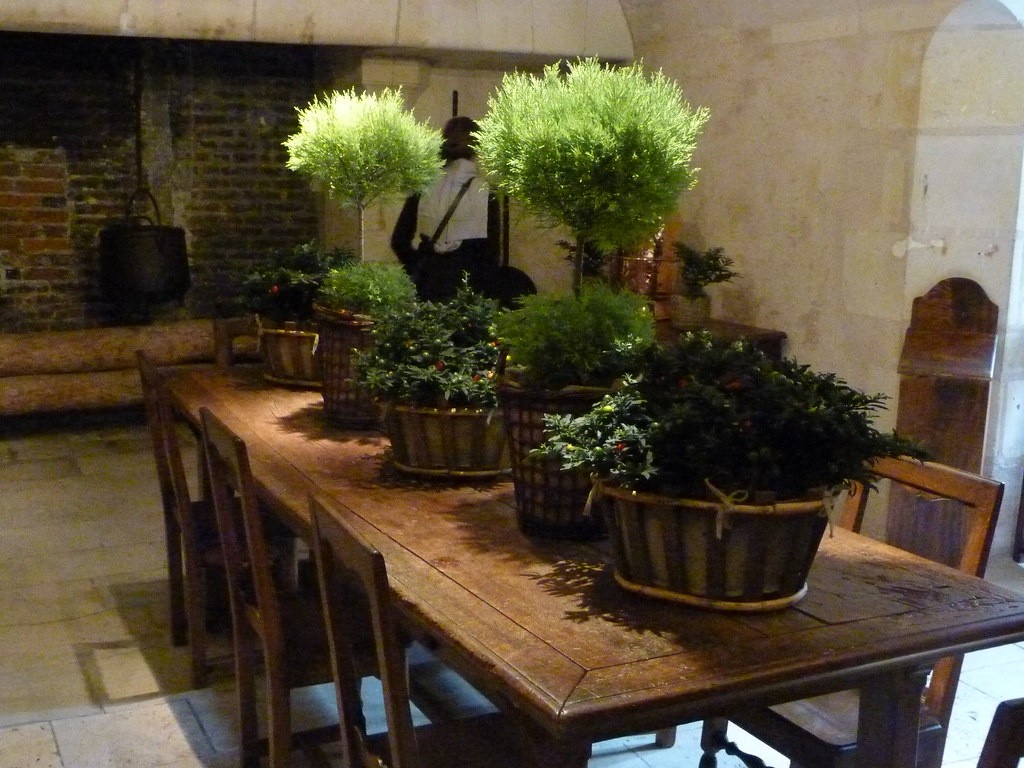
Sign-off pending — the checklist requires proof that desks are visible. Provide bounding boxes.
[156,363,1024,768]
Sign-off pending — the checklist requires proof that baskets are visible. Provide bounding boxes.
[98,189,191,311]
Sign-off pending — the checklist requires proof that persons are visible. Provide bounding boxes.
[390,116,501,289]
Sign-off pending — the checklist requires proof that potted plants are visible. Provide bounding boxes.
[667,241,738,334]
[246,250,356,389]
[353,278,514,487]
[280,88,448,433]
[559,223,670,321]
[528,335,934,613]
[468,58,713,546]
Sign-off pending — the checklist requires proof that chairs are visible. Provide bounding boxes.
[305,488,589,768]
[197,405,408,768]
[214,319,273,366]
[135,348,302,690]
[700,455,1007,768]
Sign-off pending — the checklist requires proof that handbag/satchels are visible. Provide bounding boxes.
[409,235,443,301]
